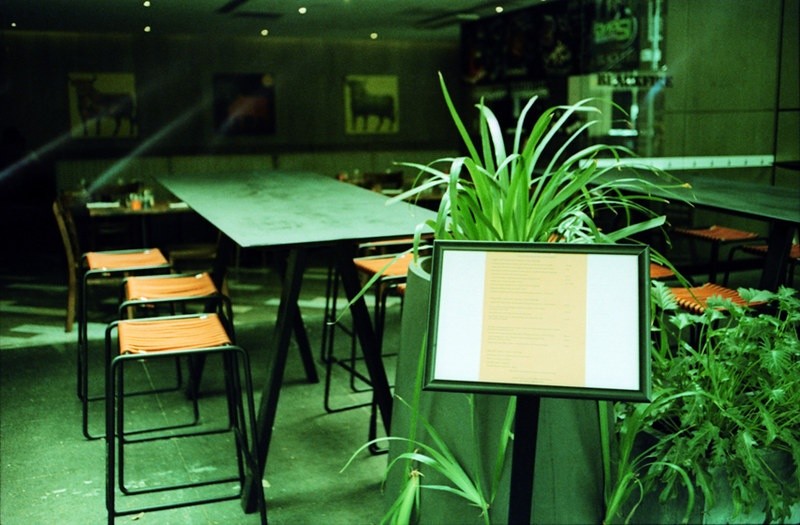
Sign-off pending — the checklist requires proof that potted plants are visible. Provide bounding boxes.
[337,71,704,525]
[613,276,800,525]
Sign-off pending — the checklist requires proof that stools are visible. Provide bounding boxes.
[719,242,800,321]
[321,247,420,456]
[77,246,200,439]
[104,312,269,525]
[649,262,700,355]
[117,269,257,497]
[673,223,768,286]
[651,280,770,373]
[317,234,427,395]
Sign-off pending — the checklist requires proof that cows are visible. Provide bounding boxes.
[343,75,397,133]
[70,73,138,138]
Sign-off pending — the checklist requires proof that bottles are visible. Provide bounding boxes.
[143,189,151,210]
[132,194,142,211]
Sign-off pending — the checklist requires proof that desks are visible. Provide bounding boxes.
[85,186,196,317]
[147,164,463,513]
[356,175,449,211]
[532,163,800,323]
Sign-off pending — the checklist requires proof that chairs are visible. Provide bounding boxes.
[53,198,140,334]
